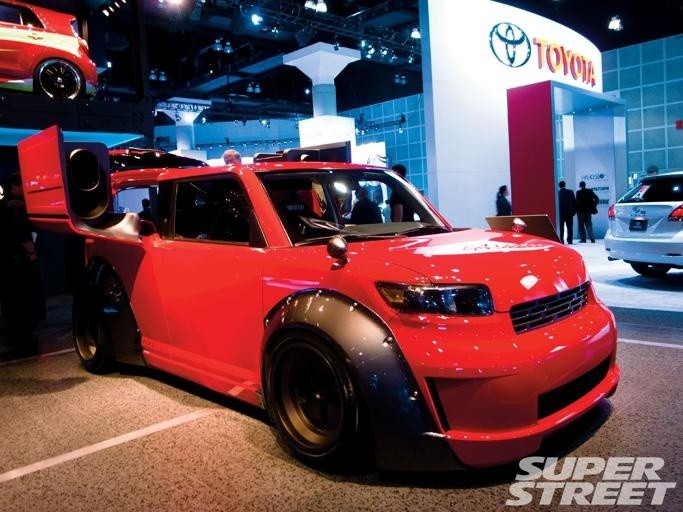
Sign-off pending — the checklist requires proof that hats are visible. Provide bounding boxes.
[18,124,619,469]
[0,1,97,104]
[604,171,683,276]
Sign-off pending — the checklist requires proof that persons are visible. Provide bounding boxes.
[0,170,40,357]
[496,186,511,216]
[559,181,576,244]
[350,187,382,224]
[383,200,392,222]
[389,164,414,222]
[576,182,599,243]
[138,199,151,218]
[223,149,241,165]
[646,166,658,177]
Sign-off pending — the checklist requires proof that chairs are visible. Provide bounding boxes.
[579,238,595,243]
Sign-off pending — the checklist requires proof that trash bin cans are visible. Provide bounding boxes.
[28,251,35,256]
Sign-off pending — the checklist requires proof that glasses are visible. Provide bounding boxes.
[175,111,180,122]
[147,39,262,94]
[304,0,327,14]
[411,28,421,40]
[395,74,408,84]
[334,41,342,50]
